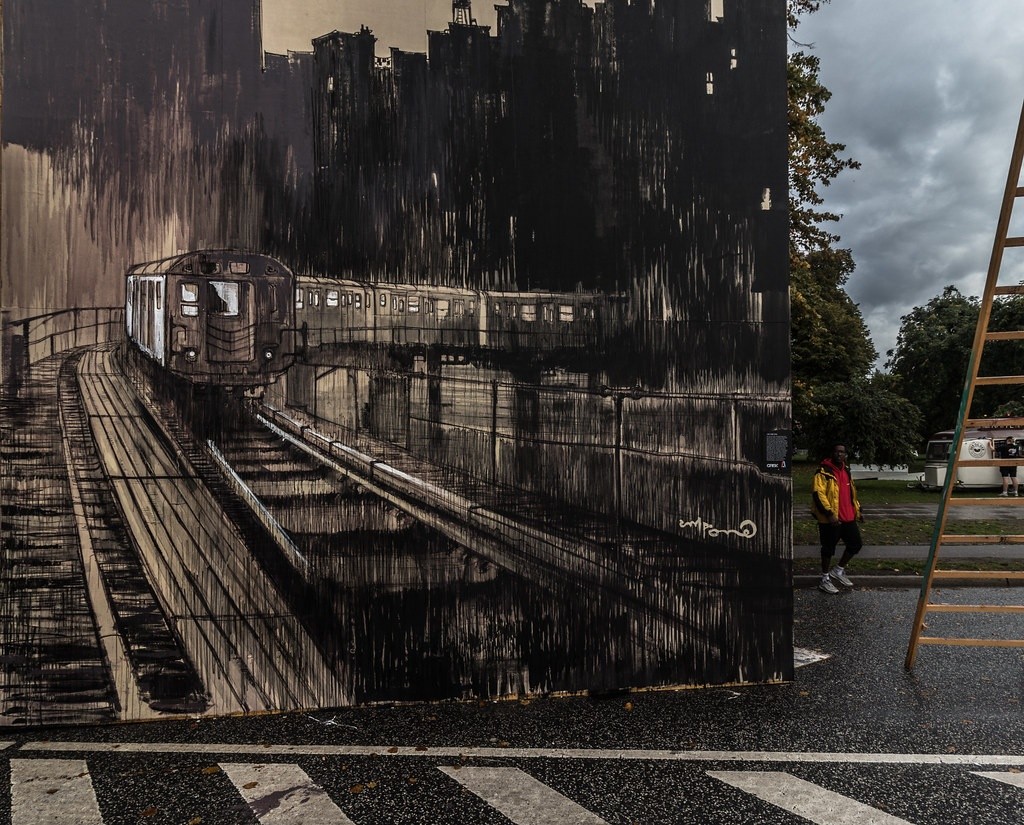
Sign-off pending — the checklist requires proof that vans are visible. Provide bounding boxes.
[922,427,1024,489]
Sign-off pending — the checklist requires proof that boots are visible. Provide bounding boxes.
[828,562,854,586]
[818,573,839,594]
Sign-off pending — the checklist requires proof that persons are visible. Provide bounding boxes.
[987,435,1022,497]
[810,441,866,596]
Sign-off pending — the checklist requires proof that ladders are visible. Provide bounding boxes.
[900,101,1024,674]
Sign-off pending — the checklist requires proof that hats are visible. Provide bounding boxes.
[1006,436,1014,440]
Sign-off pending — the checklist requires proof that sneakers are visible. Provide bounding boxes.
[997,492,1008,496]
[1014,491,1018,496]
